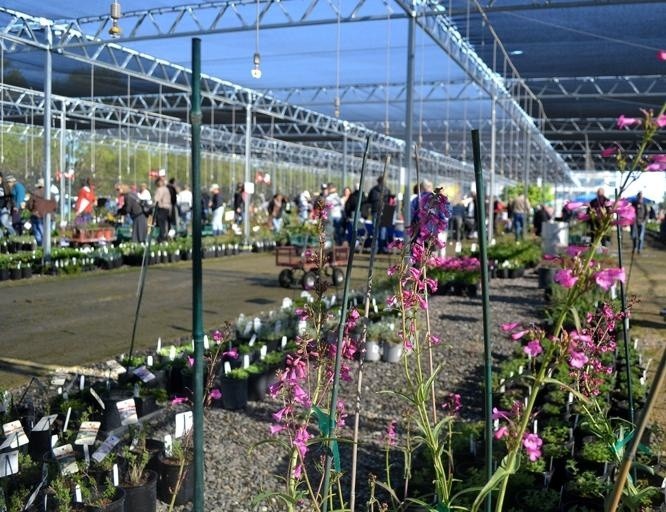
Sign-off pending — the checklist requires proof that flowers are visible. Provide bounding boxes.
[163,328,240,509]
[263,48,664,511]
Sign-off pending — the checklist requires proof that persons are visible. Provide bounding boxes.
[1,171,352,255]
[344,176,551,255]
[561,187,665,256]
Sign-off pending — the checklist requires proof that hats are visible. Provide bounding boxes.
[434,186,443,193]
[320,184,328,190]
[34,178,45,187]
[209,183,219,192]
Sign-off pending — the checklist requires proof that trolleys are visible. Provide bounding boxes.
[274,241,350,290]
[63,225,117,251]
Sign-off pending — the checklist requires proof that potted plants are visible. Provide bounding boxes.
[203,290,404,412]
[2,200,325,281]
[3,340,194,510]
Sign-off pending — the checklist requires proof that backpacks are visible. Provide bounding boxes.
[126,192,153,218]
